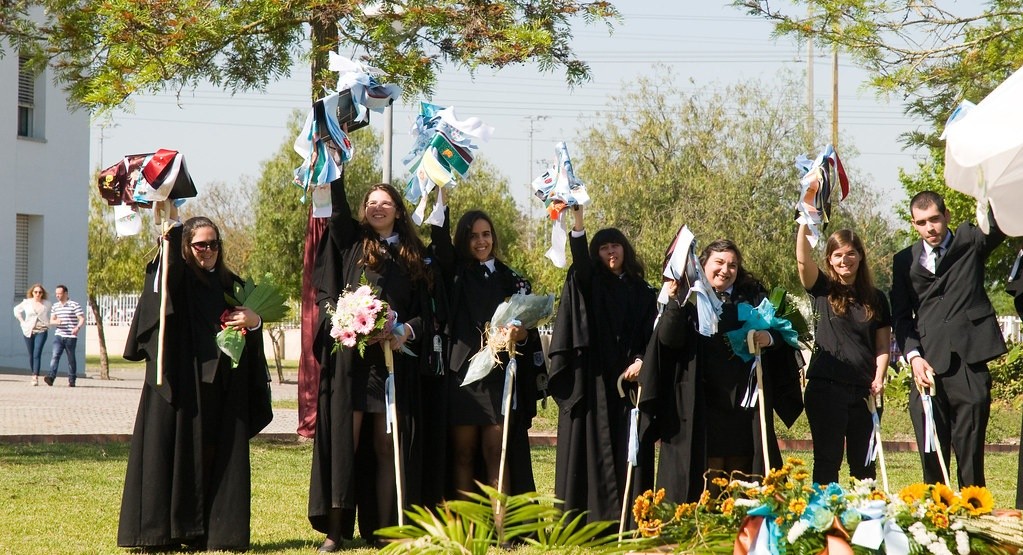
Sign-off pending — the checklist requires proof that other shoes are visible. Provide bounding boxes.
[31,379,39,386]
[69,382,75,387]
[141,543,182,553]
[44,376,54,386]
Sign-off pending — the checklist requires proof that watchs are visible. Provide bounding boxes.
[76,326,80,329]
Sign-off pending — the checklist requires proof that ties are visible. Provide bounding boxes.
[933,247,943,261]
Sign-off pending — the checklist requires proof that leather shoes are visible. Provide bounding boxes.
[366,537,390,549]
[317,532,344,552]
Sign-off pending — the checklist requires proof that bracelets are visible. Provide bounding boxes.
[247,315,261,331]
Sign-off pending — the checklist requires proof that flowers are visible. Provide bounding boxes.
[323,268,419,361]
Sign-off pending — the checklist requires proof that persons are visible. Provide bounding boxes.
[629,240,813,539]
[308,121,433,552]
[14,283,52,386]
[547,203,656,542]
[891,191,1009,492]
[1005,241,1023,510]
[44,285,85,387]
[796,174,891,485]
[117,198,274,551]
[434,184,548,549]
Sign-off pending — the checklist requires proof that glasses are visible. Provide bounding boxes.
[33,290,43,295]
[188,240,222,252]
[365,200,398,209]
